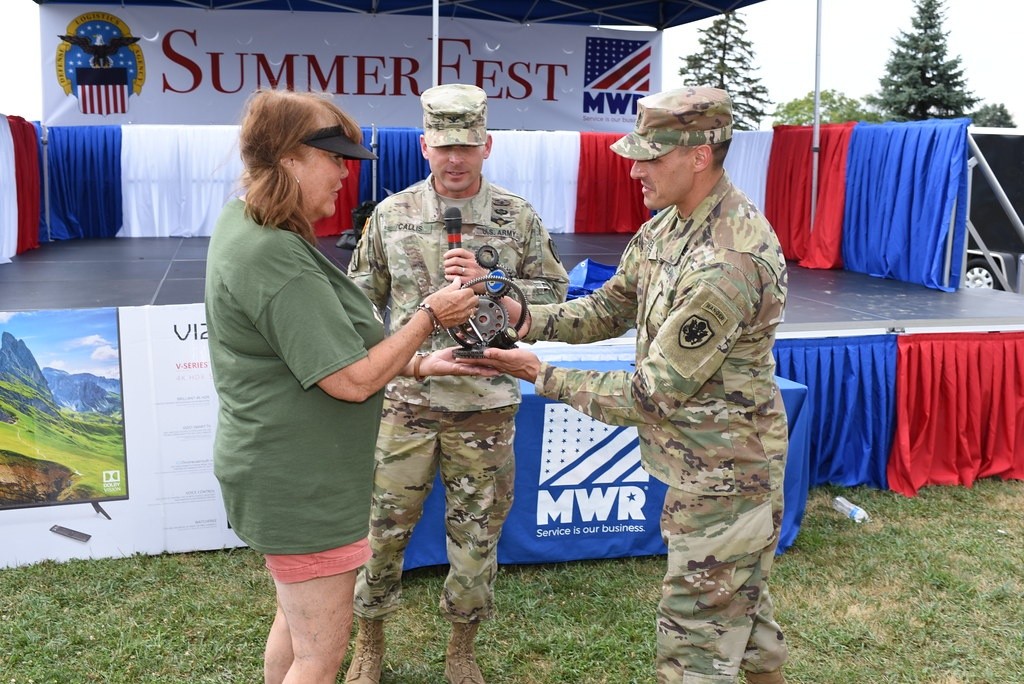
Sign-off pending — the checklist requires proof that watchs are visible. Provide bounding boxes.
[412,350,429,383]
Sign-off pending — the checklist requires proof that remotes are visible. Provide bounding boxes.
[50,525,91,542]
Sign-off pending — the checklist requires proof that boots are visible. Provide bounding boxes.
[344,616,384,684]
[444,621,486,684]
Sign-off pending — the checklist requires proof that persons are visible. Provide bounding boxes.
[339,81,569,684]
[453,85,791,684]
[203,86,500,684]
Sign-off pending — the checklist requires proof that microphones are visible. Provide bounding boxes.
[444,207,463,288]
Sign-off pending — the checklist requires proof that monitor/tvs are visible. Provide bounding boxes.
[0,307,129,520]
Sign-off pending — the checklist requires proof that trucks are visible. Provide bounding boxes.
[963,124,1023,296]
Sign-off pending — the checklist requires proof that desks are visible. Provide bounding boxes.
[405,361,807,573]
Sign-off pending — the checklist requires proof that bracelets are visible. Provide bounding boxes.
[417,302,447,345]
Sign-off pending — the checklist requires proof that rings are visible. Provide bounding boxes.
[462,268,465,273]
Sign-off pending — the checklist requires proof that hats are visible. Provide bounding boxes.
[609,85,736,161]
[299,123,380,160]
[421,84,488,147]
